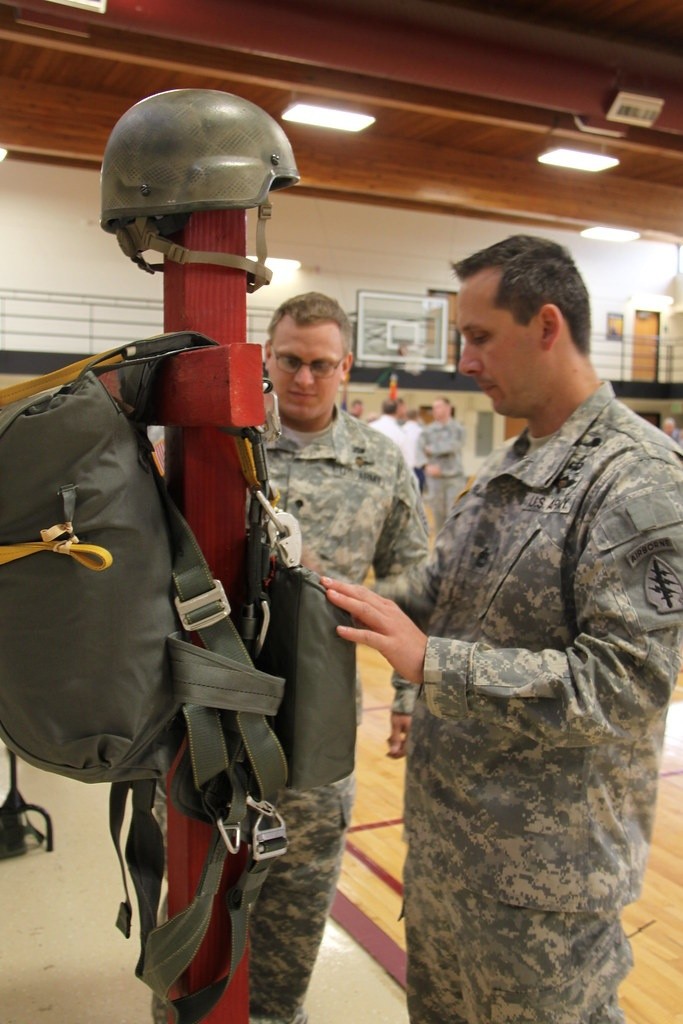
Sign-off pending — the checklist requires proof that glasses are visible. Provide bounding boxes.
[268,342,350,379]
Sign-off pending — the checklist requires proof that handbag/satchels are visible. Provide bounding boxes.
[267,551,358,791]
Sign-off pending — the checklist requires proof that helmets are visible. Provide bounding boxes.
[99,87,304,237]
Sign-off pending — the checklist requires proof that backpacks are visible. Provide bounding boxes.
[0,330,289,1024]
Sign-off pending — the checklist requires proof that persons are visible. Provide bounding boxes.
[314,230,683,1024]
[246,291,433,1024]
[344,393,466,529]
[661,415,683,447]
